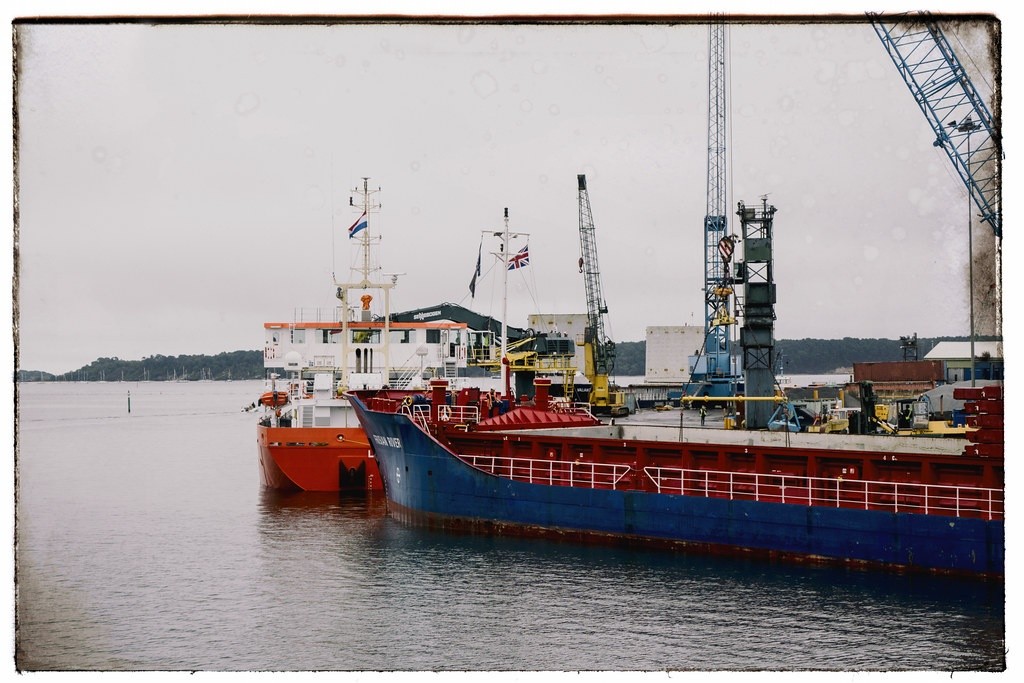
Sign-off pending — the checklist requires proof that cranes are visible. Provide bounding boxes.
[680,19,746,412]
[870,19,999,237]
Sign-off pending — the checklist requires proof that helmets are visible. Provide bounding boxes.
[491,386,496,390]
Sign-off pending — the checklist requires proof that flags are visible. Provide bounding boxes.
[506,244,529,271]
[348,210,368,240]
[469,241,481,298]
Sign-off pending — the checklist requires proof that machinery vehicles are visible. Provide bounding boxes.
[577,173,630,417]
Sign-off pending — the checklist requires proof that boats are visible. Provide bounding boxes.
[343,191,1004,595]
[244,176,519,493]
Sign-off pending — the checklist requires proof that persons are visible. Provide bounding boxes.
[699,405,706,426]
[901,405,912,428]
[481,387,504,418]
[780,411,787,431]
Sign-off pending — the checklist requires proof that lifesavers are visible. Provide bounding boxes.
[440,407,452,422]
